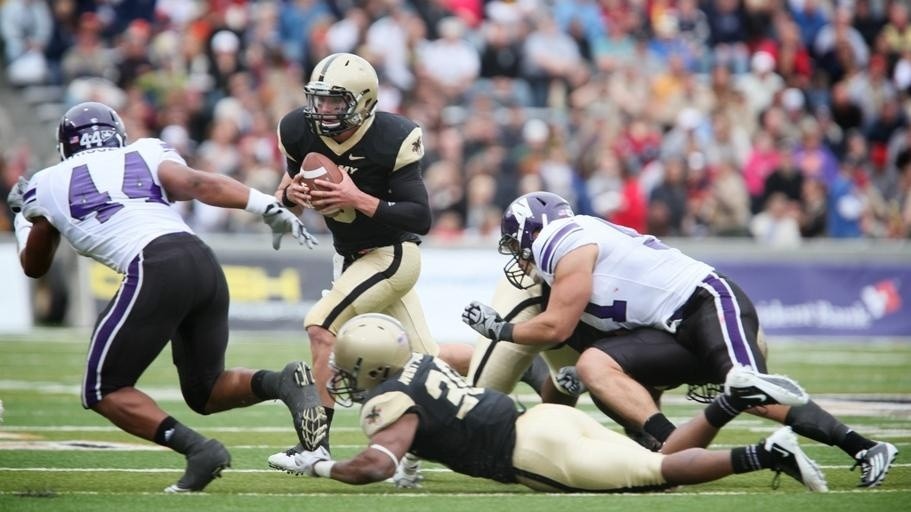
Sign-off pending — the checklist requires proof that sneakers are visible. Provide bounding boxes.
[269,443,333,472]
[765,424,829,494]
[164,439,232,496]
[854,442,898,490]
[724,364,809,409]
[280,361,329,450]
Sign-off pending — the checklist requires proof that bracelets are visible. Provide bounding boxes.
[281,184,299,209]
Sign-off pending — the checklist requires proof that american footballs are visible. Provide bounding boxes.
[297,152,341,218]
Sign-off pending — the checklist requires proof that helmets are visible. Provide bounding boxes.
[55,102,127,159]
[333,312,412,404]
[308,53,379,136]
[500,191,575,285]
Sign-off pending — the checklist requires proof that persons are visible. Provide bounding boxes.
[8,97,333,494]
[285,309,830,495]
[385,264,771,490]
[267,49,579,477]
[0,1,206,247]
[583,1,911,246]
[459,191,900,490]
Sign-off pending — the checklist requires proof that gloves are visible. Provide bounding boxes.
[6,175,33,254]
[556,366,587,397]
[462,300,515,343]
[244,187,319,251]
